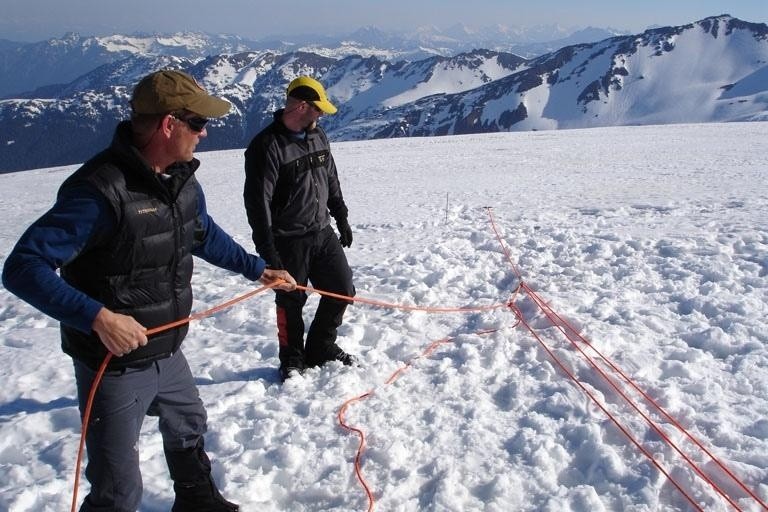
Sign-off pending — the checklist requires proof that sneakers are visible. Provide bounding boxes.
[279,364,302,382]
[306,350,351,367]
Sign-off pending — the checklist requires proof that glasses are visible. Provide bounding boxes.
[174,115,208,132]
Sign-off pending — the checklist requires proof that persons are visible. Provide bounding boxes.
[243,76,355,384]
[0,69,300,512]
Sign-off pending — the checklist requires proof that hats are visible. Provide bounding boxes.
[287,76,337,114]
[129,70,231,118]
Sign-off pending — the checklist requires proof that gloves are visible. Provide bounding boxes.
[337,216,352,248]
[260,246,284,270]
